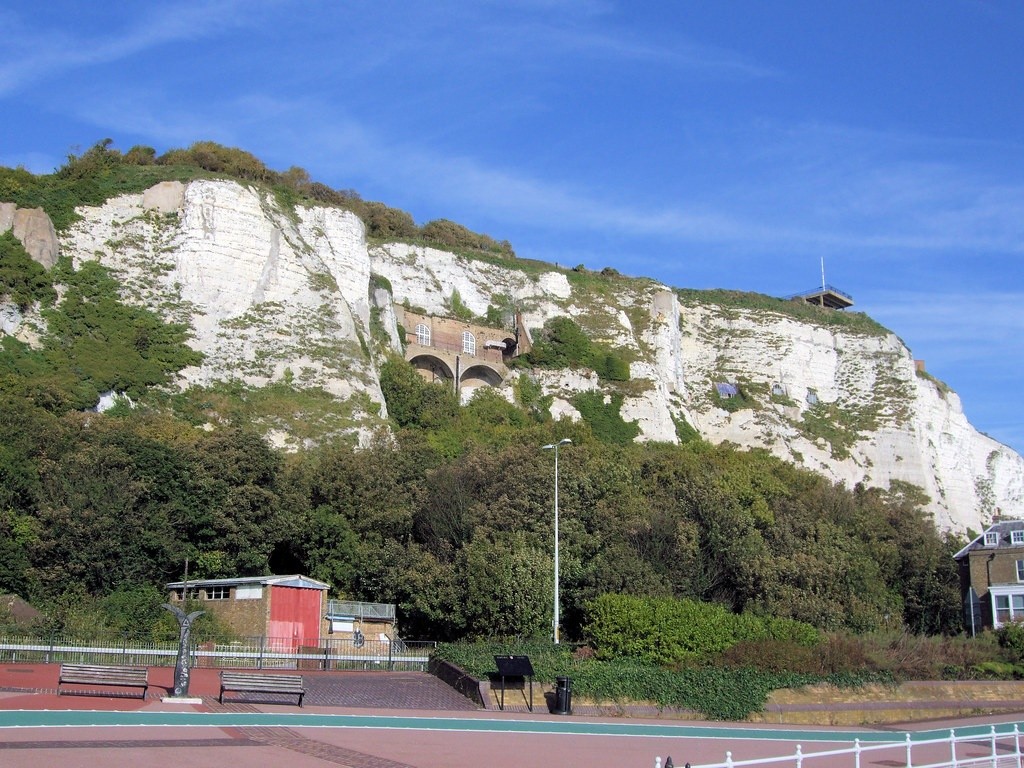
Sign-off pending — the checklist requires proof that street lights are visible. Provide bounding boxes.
[543,437,572,645]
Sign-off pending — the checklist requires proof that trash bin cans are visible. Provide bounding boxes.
[554,675,573,715]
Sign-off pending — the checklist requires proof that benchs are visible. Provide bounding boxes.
[218,671,306,708]
[57,661,149,703]
